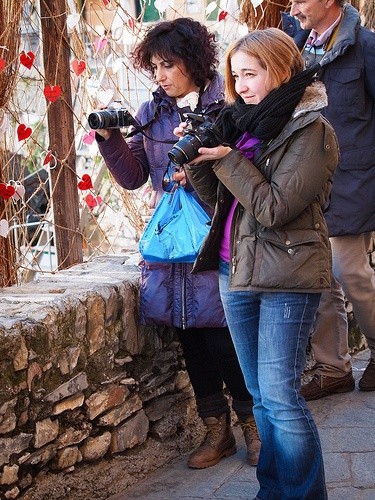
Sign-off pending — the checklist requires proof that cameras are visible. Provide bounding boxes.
[166,111,226,168]
[87,98,133,131]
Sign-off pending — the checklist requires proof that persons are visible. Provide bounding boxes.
[280,0,375,401]
[167,27,341,500]
[88,17,261,469]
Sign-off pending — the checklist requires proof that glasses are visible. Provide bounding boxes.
[305,45,317,69]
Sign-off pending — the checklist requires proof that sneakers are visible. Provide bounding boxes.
[300,368,356,401]
[358,358,375,392]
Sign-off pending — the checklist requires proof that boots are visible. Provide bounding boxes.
[233,415,262,466]
[187,410,238,468]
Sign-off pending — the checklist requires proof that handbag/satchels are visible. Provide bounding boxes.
[138,180,213,264]
